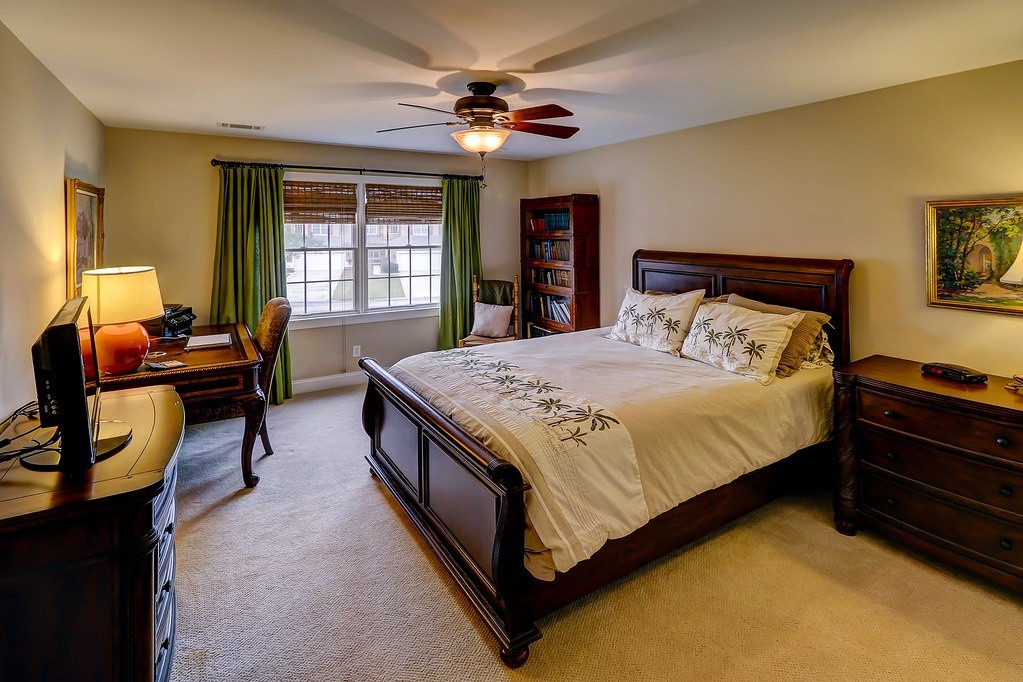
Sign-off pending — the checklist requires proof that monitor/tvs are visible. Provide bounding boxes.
[19,296,133,473]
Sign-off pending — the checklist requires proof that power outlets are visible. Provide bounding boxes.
[353,345,361,358]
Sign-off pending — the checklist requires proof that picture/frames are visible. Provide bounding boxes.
[66,177,106,300]
[924,195,1023,316]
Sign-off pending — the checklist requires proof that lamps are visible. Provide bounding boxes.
[450,122,511,189]
[82,265,165,377]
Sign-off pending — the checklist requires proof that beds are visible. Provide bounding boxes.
[358,249,856,670]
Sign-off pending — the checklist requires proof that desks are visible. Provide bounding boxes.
[100,321,267,489]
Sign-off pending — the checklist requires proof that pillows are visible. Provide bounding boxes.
[680,302,806,386]
[726,293,831,379]
[603,285,706,360]
[644,289,729,303]
[470,302,514,338]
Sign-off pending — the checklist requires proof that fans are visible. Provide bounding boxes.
[376,81,580,140]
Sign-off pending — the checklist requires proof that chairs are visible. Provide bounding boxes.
[254,296,292,455]
[458,274,519,348]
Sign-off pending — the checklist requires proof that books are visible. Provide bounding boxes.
[528,212,570,338]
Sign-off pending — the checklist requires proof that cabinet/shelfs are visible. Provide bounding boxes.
[519,194,600,339]
[0,384,186,682]
[832,354,1023,596]
